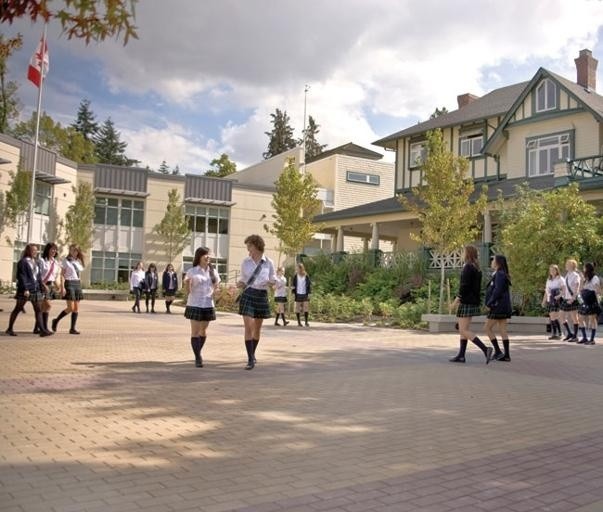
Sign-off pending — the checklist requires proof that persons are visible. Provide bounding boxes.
[144,263,158,313]
[129,262,146,313]
[450,246,493,363]
[184,246,221,368]
[482,253,512,362]
[227,234,276,369]
[6,243,86,336]
[163,263,178,314]
[272,267,289,326]
[291,263,312,327]
[541,259,602,345]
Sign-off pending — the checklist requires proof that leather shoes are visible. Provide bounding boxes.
[33,318,57,336]
[243,361,254,370]
[449,357,466,362]
[485,347,511,364]
[4,328,16,336]
[195,356,203,367]
[548,332,595,345]
[70,328,79,333]
